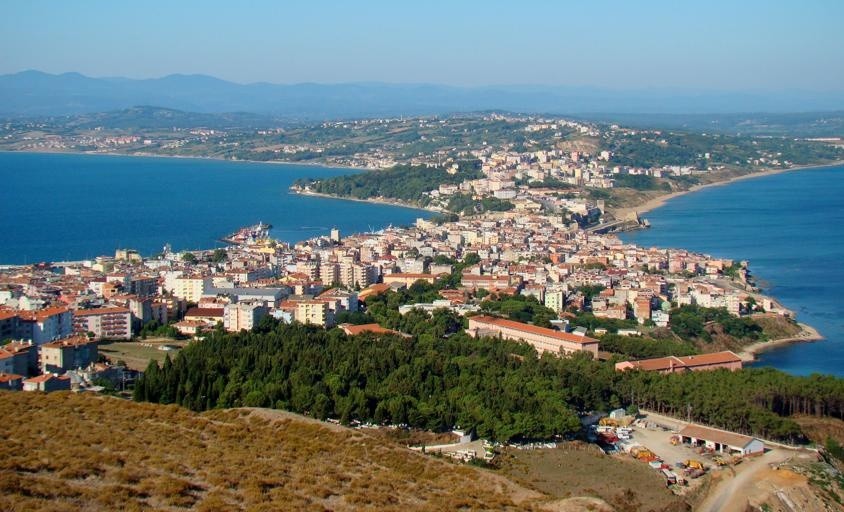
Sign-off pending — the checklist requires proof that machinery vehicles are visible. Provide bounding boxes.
[593,424,740,485]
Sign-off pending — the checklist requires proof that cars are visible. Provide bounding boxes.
[448,447,495,462]
